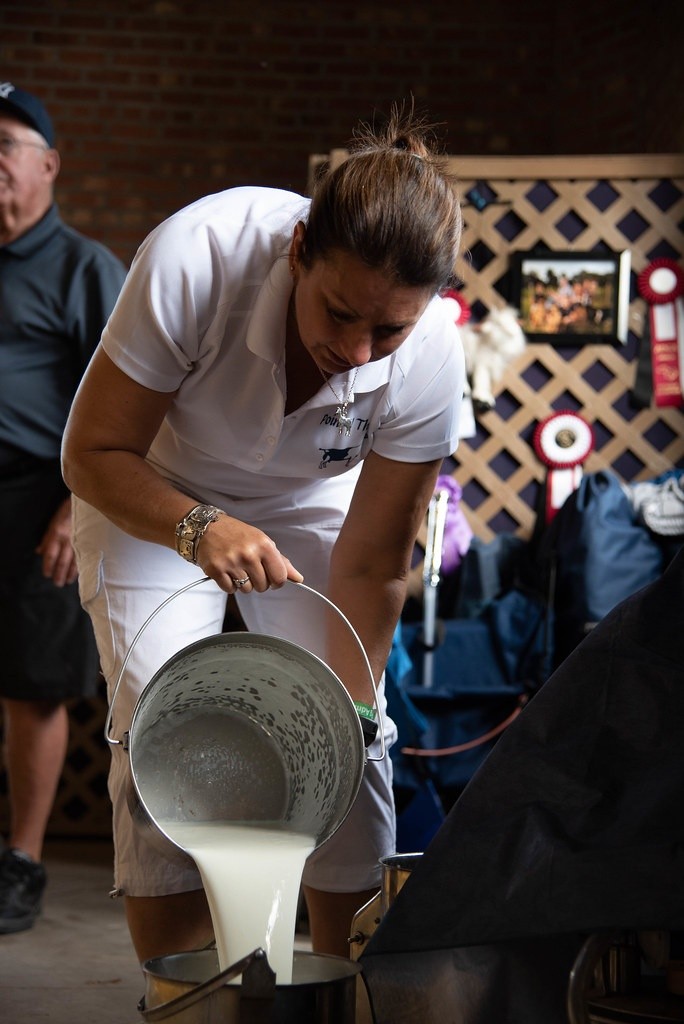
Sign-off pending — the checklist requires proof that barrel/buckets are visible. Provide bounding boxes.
[137,940,361,1023]
[104,576,385,856]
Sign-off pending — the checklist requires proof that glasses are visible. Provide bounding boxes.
[1,137,47,157]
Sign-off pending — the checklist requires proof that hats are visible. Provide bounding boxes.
[0,81,53,148]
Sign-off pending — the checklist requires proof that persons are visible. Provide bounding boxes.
[0,81,127,934]
[528,277,594,329]
[56,126,461,968]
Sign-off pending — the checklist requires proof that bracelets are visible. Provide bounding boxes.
[174,503,223,564]
[354,701,377,721]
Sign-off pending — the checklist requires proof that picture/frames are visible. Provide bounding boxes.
[509,250,630,345]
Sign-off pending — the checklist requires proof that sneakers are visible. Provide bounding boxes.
[0,852,46,935]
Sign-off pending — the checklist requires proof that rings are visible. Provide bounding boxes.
[233,577,250,588]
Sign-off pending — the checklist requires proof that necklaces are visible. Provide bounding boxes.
[313,360,359,437]
